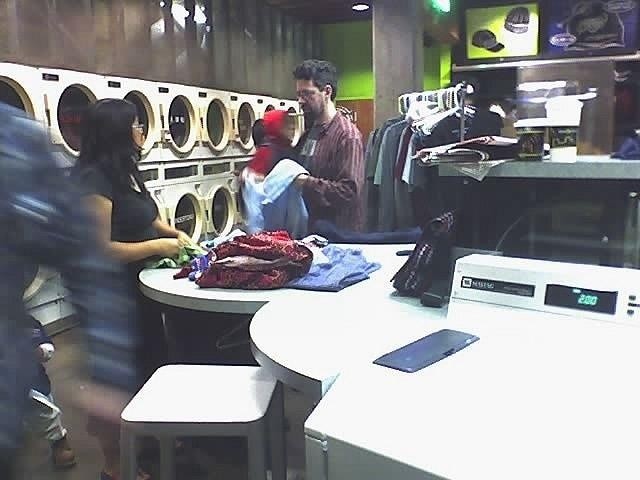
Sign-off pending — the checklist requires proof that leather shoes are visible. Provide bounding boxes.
[50,434,76,468]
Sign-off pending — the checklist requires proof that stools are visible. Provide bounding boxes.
[119,365,288,479]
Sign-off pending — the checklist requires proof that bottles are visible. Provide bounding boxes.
[544,95,583,163]
[513,118,546,161]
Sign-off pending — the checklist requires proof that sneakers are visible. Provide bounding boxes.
[102,463,151,479]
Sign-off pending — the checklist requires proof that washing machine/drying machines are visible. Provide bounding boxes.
[164,176,202,245]
[280,99,301,148]
[199,89,230,160]
[160,82,200,162]
[105,76,160,163]
[23,264,62,308]
[145,181,164,224]
[46,68,105,161]
[0,62,46,125]
[259,95,280,120]
[202,172,237,240]
[231,92,258,158]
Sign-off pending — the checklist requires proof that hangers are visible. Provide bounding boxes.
[397,82,474,118]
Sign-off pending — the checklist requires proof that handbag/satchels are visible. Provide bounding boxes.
[390,212,457,297]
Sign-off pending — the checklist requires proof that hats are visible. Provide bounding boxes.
[504,7,529,34]
[471,30,504,52]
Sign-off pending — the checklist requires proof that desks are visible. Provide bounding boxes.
[438,147,640,269]
[135,243,640,479]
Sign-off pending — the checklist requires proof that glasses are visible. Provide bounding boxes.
[132,123,145,134]
[297,90,316,100]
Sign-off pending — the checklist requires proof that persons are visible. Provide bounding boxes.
[413,73,506,250]
[59,99,198,480]
[291,59,367,234]
[14,312,77,467]
[230,119,264,177]
[0,99,140,480]
[240,110,299,241]
